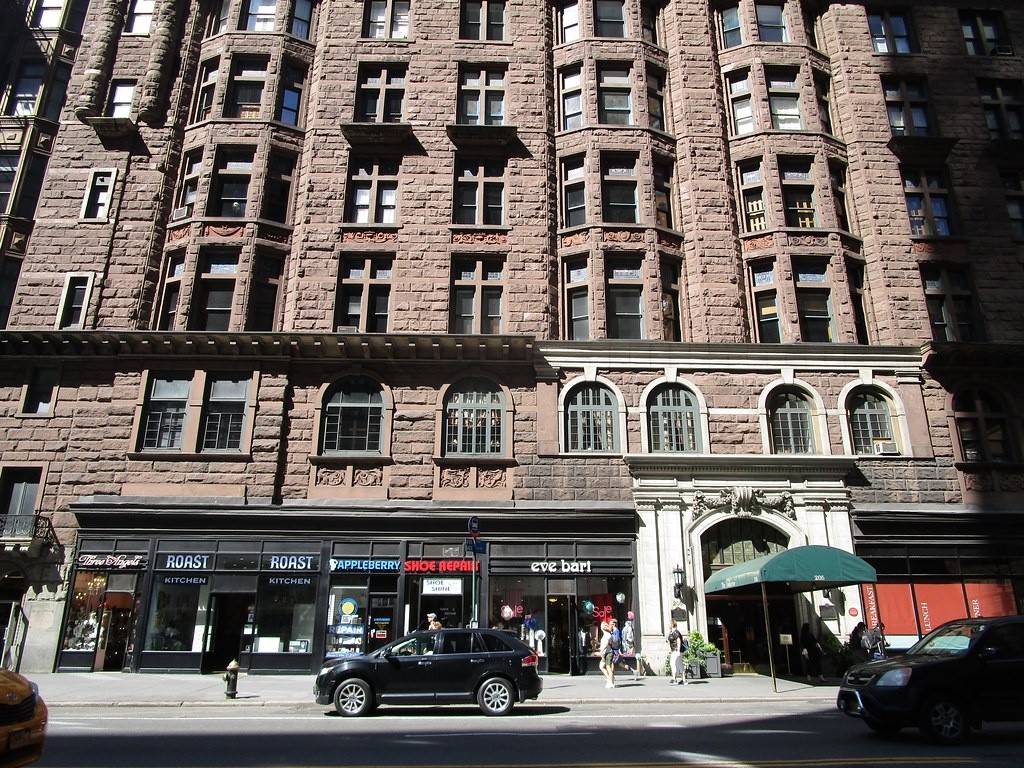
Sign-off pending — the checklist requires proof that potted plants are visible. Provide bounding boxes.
[663,630,722,679]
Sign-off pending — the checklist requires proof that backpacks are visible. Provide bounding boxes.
[680,640,688,652]
[861,629,881,650]
[609,633,620,650]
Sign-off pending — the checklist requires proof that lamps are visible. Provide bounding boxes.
[672,563,685,598]
[823,589,829,598]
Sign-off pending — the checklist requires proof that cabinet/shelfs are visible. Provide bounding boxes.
[326,624,364,646]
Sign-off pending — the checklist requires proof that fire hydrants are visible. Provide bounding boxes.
[222,658,240,699]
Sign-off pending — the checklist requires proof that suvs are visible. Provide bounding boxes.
[312,627,544,718]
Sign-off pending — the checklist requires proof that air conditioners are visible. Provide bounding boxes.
[874,441,898,455]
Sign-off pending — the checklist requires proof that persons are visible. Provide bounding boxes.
[402,613,441,655]
[668,619,689,685]
[799,622,827,682]
[599,619,638,688]
[847,622,891,663]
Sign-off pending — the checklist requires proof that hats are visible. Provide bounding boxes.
[427,613,437,619]
[534,630,546,640]
[858,622,867,628]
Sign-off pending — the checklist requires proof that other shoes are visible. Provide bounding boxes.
[633,670,638,682]
[679,680,688,685]
[604,683,616,688]
[819,678,826,682]
[668,680,678,685]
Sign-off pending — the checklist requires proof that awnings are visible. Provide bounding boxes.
[705,544,877,693]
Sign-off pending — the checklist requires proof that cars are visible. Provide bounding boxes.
[0,668,48,768]
[836,615,1024,746]
